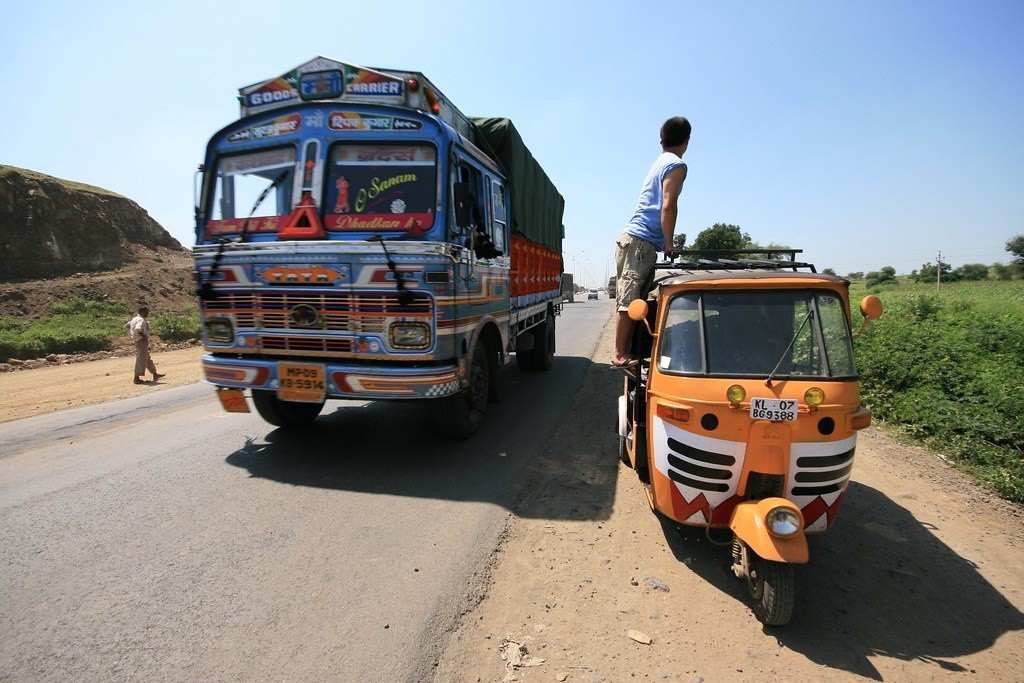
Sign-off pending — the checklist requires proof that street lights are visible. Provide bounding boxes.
[563,250,590,294]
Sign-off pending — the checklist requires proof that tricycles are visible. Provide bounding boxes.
[616,246,884,627]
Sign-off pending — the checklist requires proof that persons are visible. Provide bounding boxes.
[610,117,691,369]
[123,307,166,384]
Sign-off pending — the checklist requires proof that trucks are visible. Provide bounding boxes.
[608,276,617,299]
[561,272,575,304]
[191,54,569,441]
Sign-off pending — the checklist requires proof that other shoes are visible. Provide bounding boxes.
[153,373,166,380]
[610,355,639,370]
[133,378,144,384]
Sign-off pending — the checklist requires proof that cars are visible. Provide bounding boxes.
[587,288,599,301]
